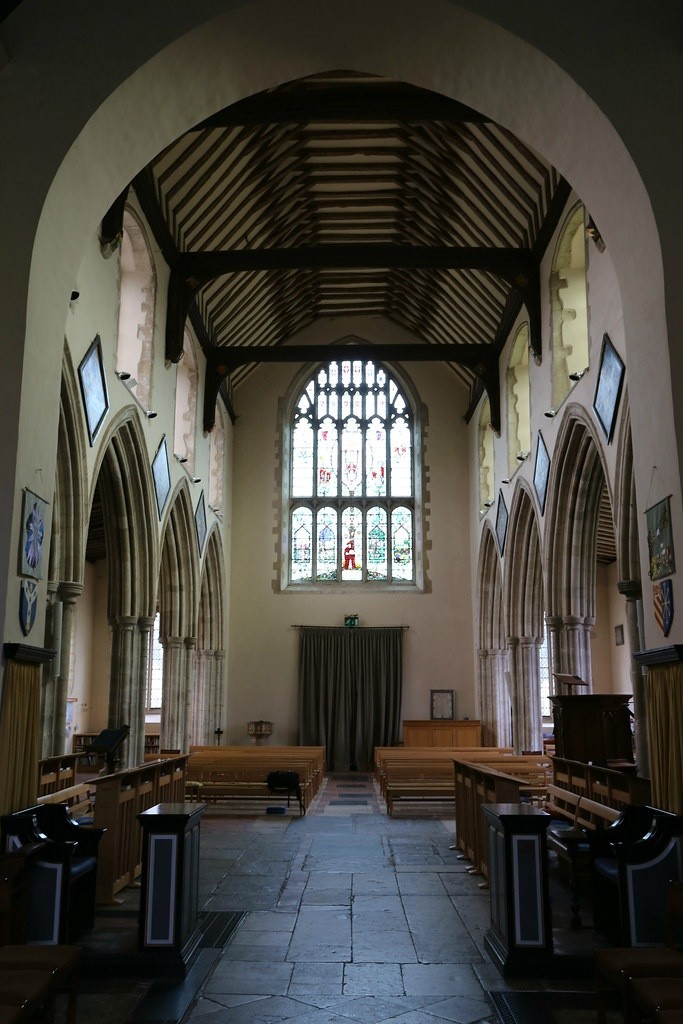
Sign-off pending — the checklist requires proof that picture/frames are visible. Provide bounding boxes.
[533,430,551,517]
[193,489,207,559]
[151,434,171,521]
[78,335,109,447]
[495,488,508,558]
[591,333,626,447]
[431,690,455,719]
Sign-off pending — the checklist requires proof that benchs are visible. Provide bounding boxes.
[38,782,94,825]
[186,746,325,815]
[544,784,621,865]
[373,746,555,816]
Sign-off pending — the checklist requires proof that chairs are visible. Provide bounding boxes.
[0,802,108,1024]
[565,800,683,1024]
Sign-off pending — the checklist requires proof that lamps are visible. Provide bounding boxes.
[570,371,583,380]
[484,479,509,507]
[517,450,527,461]
[178,457,201,483]
[117,372,158,419]
[71,291,79,300]
[544,410,555,417]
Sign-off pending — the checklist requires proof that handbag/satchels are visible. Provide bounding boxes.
[267,771,299,792]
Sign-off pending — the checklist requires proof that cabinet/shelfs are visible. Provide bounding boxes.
[403,720,482,747]
[72,733,160,774]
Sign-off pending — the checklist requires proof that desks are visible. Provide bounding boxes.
[135,803,205,983]
[481,803,555,976]
